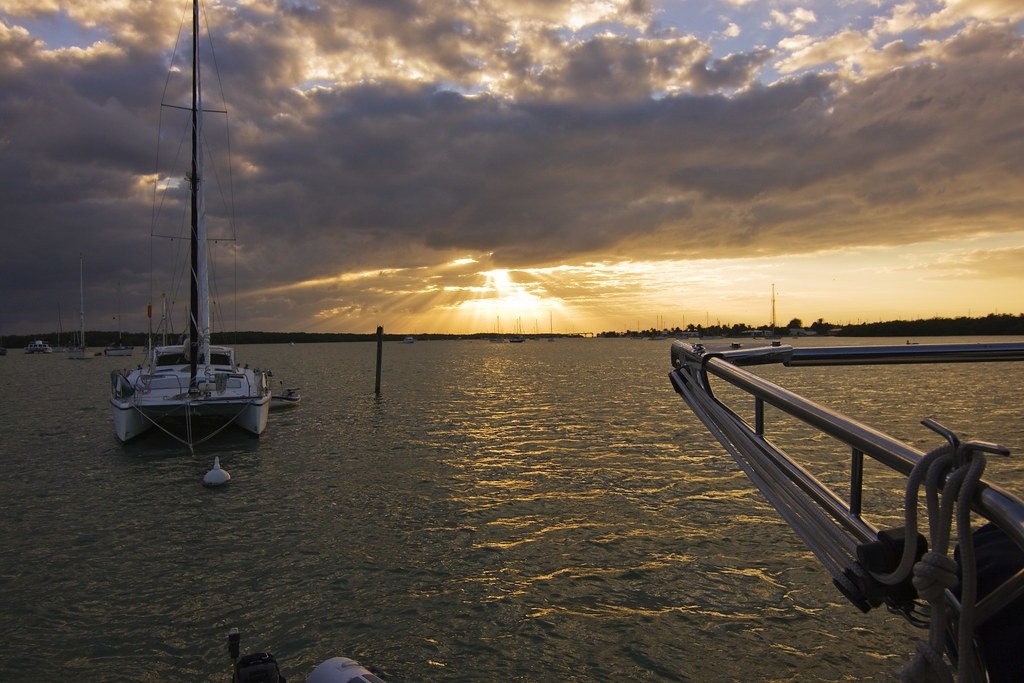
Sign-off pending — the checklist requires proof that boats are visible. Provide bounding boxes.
[24,340,52,354]
[268,381,301,412]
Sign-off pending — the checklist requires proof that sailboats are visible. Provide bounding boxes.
[53,253,94,359]
[104,282,135,357]
[109,0,272,442]
